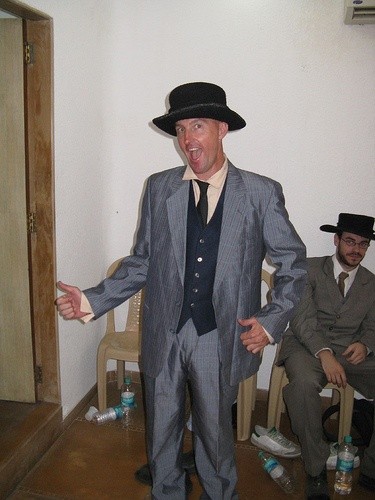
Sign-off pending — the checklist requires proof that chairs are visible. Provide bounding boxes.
[97,258,145,412]
[236,270,353,446]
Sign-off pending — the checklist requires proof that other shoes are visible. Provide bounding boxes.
[135,463,194,494]
[178,450,198,473]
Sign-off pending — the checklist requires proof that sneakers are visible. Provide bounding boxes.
[325,441,360,470]
[306,467,331,500]
[356,472,375,494]
[251,424,303,459]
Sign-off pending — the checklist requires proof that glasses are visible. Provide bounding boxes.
[341,239,371,249]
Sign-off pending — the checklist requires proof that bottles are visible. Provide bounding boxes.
[120,377,136,426]
[334,435,355,496]
[92,402,140,426]
[257,451,298,494]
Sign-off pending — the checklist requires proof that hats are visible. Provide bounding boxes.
[151,82,246,137]
[319,212,375,240]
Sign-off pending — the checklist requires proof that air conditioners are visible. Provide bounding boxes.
[344,0,375,25]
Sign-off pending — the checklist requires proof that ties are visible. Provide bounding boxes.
[337,272,349,297]
[195,180,210,225]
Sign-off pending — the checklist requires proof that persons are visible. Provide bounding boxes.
[56,81,308,500]
[276,212,375,500]
